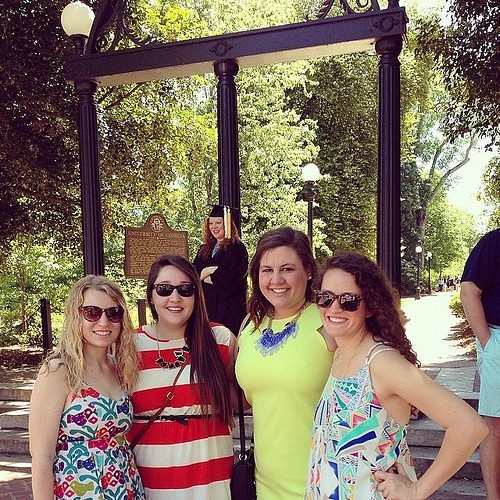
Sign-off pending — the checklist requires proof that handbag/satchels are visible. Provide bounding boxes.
[229,446,257,500]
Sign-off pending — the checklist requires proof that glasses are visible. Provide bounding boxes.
[313,289,368,312]
[152,283,196,298]
[78,306,125,323]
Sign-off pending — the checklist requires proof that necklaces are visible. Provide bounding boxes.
[266,301,309,338]
[155,322,189,369]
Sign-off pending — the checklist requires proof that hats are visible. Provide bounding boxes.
[205,204,239,239]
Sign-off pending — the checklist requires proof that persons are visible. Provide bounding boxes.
[233,227,338,500]
[461,227,500,500]
[306,253,489,500]
[439,276,458,292]
[194,205,248,336]
[106,255,235,500]
[28,277,147,500]
